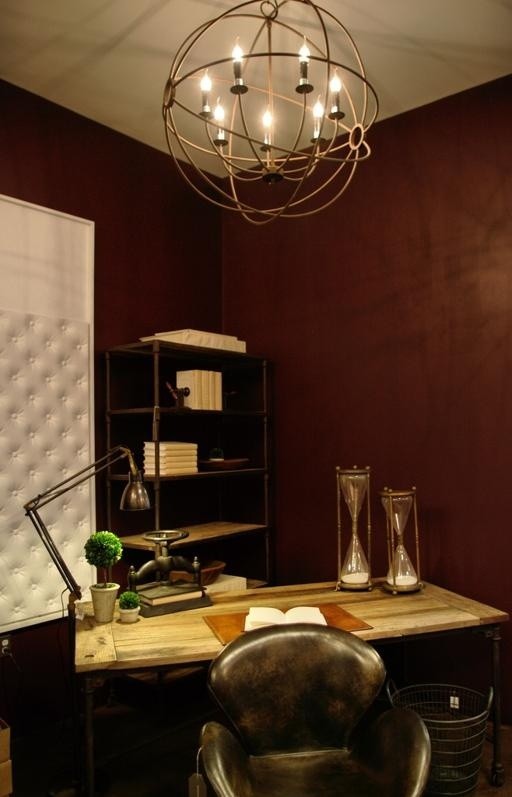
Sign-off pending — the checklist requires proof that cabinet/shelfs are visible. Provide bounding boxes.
[104,339,271,581]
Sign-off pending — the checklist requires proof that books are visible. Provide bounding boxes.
[126,576,207,608]
[242,604,328,634]
[141,440,200,476]
[175,370,225,411]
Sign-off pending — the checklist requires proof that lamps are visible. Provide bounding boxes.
[23,446,151,616]
[161,0,379,224]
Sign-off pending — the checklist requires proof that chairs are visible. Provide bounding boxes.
[201,623,432,797]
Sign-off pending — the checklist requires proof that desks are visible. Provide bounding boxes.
[74,576,510,797]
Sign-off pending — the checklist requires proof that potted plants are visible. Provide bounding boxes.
[117,591,141,623]
[84,531,123,622]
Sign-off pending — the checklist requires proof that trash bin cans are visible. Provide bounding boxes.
[387,681,494,797]
